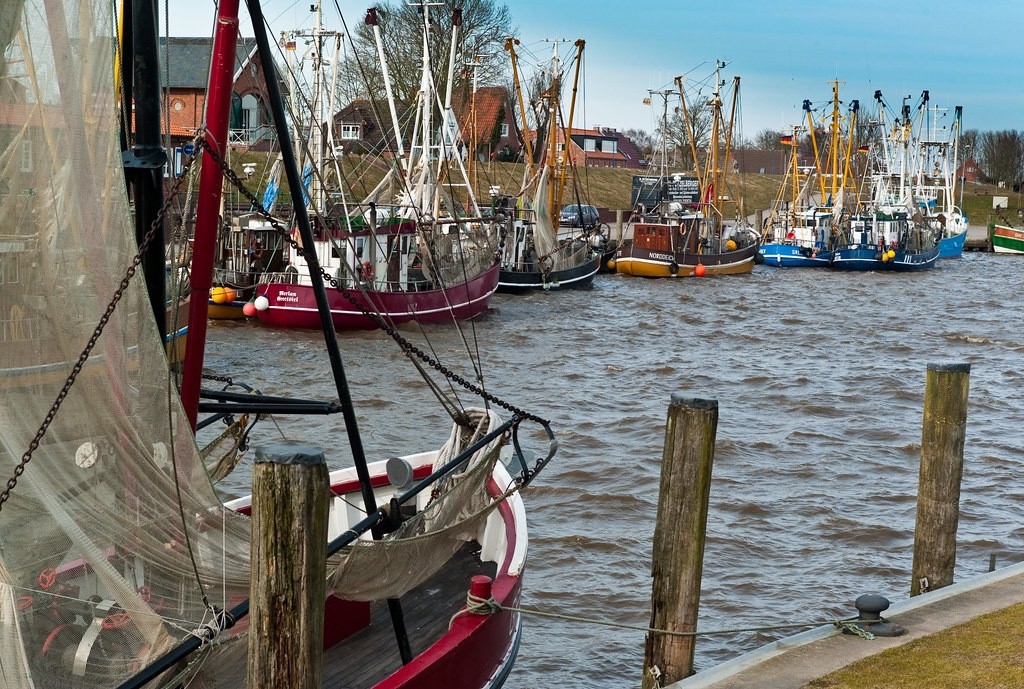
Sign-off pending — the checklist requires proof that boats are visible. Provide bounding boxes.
[755,76,970,272]
[0,0,557,689]
[988,184,1024,255]
[156,0,608,330]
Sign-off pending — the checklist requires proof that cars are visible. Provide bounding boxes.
[559,204,599,228]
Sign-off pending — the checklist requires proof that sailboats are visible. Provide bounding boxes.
[615,57,760,280]
